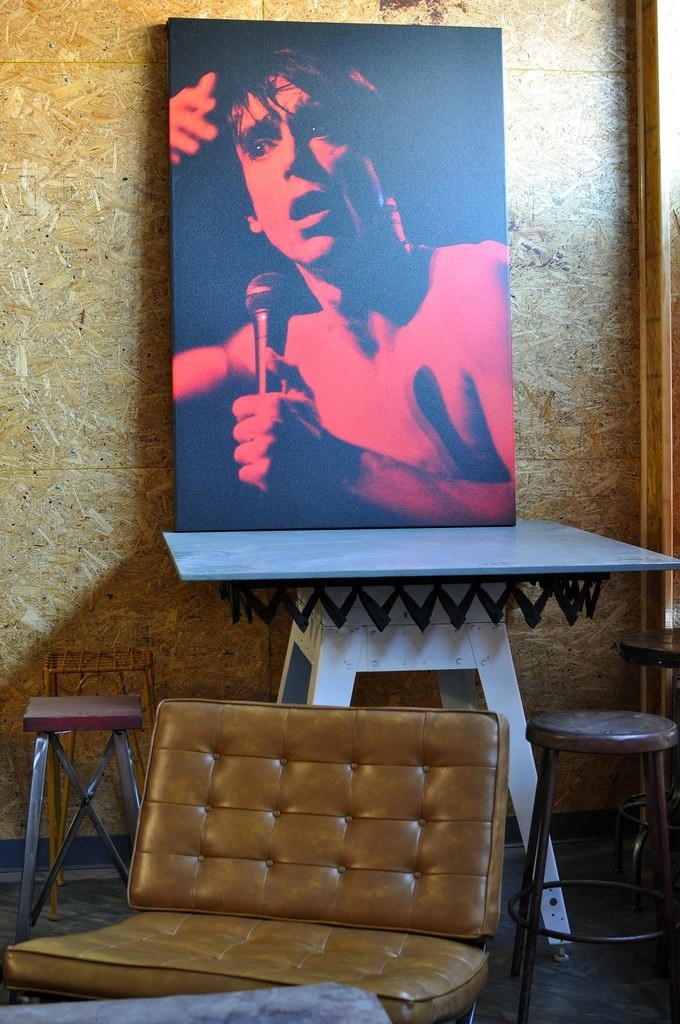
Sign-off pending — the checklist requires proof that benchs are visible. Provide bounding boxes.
[3,697,507,1024]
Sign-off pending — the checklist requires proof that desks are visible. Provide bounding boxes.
[163,520,680,961]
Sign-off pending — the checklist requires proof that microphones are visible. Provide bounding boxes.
[245,271,296,396]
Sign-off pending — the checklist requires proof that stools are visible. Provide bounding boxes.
[508,710,680,1024]
[615,628,680,911]
[43,647,153,920]
[9,695,142,1005]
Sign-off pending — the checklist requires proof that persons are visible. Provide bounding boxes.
[170,46,515,527]
